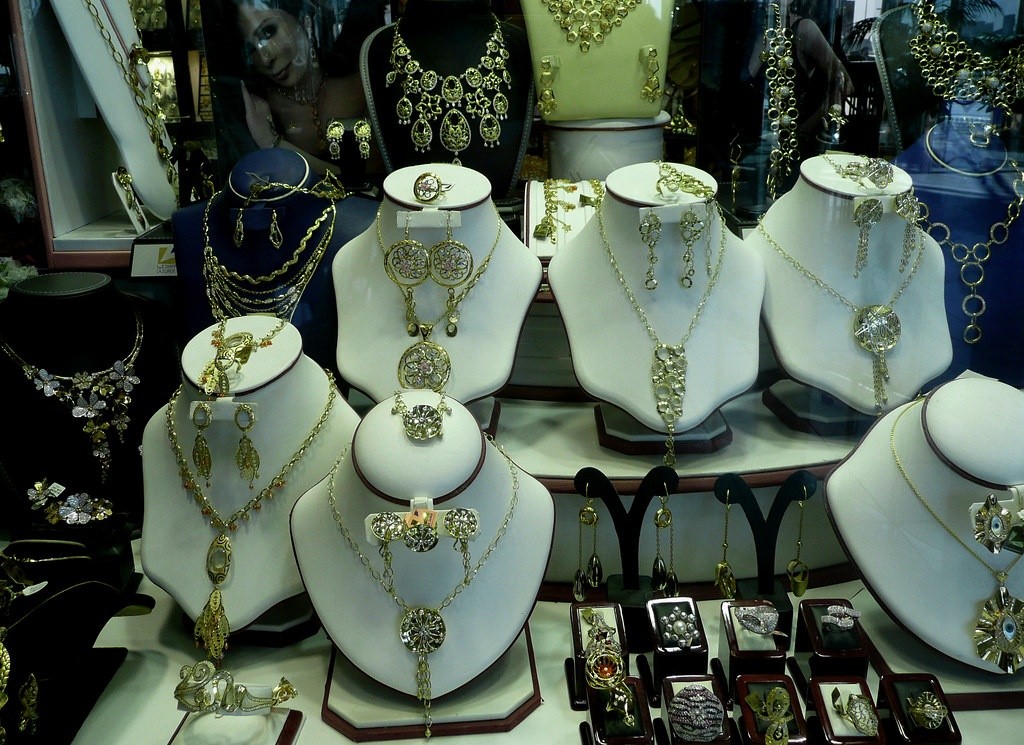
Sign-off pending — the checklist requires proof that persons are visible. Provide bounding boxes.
[239,0,386,187]
[745,0,853,157]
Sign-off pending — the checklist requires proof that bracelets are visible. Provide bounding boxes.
[174,660,296,716]
[265,113,281,149]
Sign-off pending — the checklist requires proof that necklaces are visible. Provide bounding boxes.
[328,431,519,738]
[280,77,328,148]
[199,170,346,350]
[87,0,179,199]
[0,549,122,708]
[596,159,728,466]
[913,158,1024,344]
[375,201,502,392]
[389,17,509,162]
[755,155,925,416]
[0,317,146,481]
[903,0,1024,147]
[890,395,1024,676]
[166,366,337,662]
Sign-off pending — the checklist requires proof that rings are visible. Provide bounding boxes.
[908,692,948,729]
[832,688,878,736]
[660,607,699,649]
[746,687,794,745]
[668,684,724,742]
[582,609,636,728]
[736,606,779,634]
[821,605,860,630]
